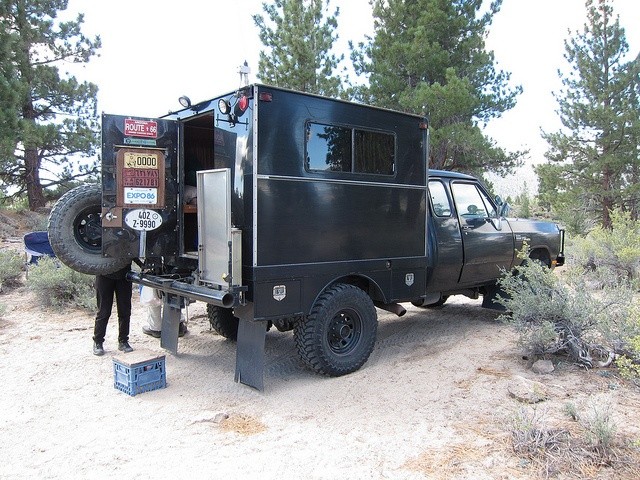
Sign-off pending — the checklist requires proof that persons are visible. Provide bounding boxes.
[92,258,145,356]
[139,284,189,340]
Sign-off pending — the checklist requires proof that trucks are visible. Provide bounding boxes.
[103,83,564,390]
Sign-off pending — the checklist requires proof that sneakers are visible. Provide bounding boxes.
[179,322,187,337]
[93,341,103,355]
[119,343,133,352]
[142,326,161,337]
